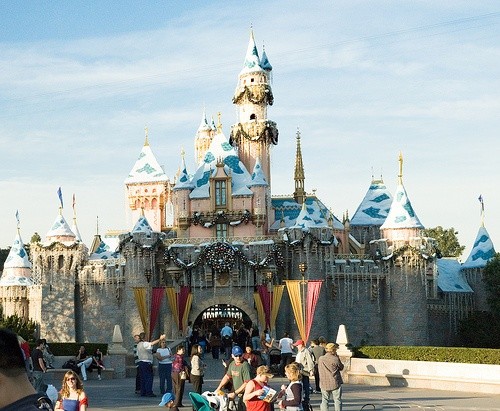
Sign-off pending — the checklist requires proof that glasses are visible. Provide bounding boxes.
[66,377,76,380]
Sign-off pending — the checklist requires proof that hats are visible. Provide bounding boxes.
[159,393,175,406]
[292,340,304,346]
[232,346,243,356]
[325,342,339,351]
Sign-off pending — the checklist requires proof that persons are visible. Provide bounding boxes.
[292,336,328,393]
[0,327,54,411]
[31,339,54,390]
[54,370,88,411]
[76,345,93,382]
[277,362,304,411]
[91,348,105,381]
[132,332,205,408]
[318,342,344,411]
[159,393,179,411]
[185,320,294,411]
[243,364,274,411]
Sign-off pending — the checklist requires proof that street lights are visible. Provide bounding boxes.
[173,272,183,331]
[266,270,274,338]
[299,261,309,347]
[144,267,153,341]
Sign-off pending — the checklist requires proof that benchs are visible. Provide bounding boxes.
[43,368,115,380]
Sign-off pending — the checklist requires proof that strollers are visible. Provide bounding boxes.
[189,390,235,411]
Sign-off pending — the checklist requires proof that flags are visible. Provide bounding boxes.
[15,211,20,225]
[478,195,484,211]
[72,195,76,208]
[57,188,64,208]
